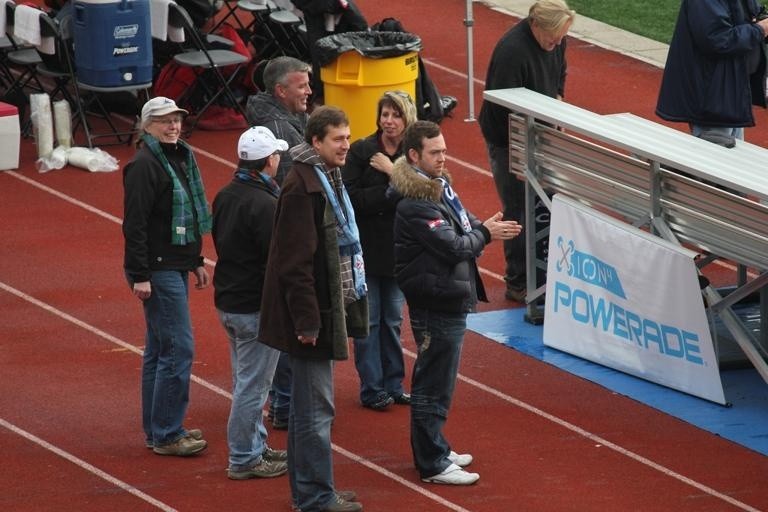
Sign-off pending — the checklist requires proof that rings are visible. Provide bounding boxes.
[504,229,508,233]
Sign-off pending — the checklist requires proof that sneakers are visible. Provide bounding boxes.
[227,460,291,480]
[396,391,415,405]
[447,450,473,466]
[146,428,203,449]
[505,286,545,306]
[263,447,289,461]
[293,490,356,509]
[323,494,363,511]
[421,463,480,484]
[267,405,289,430]
[367,393,393,409]
[152,435,209,455]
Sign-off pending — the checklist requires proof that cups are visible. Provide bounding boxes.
[29,94,101,174]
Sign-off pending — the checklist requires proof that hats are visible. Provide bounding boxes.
[237,125,289,162]
[141,96,189,124]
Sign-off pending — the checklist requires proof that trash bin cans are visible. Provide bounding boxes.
[313,31,422,148]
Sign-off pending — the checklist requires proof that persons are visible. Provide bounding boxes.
[211,125,290,479]
[342,90,418,408]
[252,56,314,430]
[384,120,523,485]
[258,105,371,512]
[655,0,768,141]
[478,0,575,303]
[121,96,211,456]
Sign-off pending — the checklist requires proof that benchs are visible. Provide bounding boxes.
[481,68,768,380]
